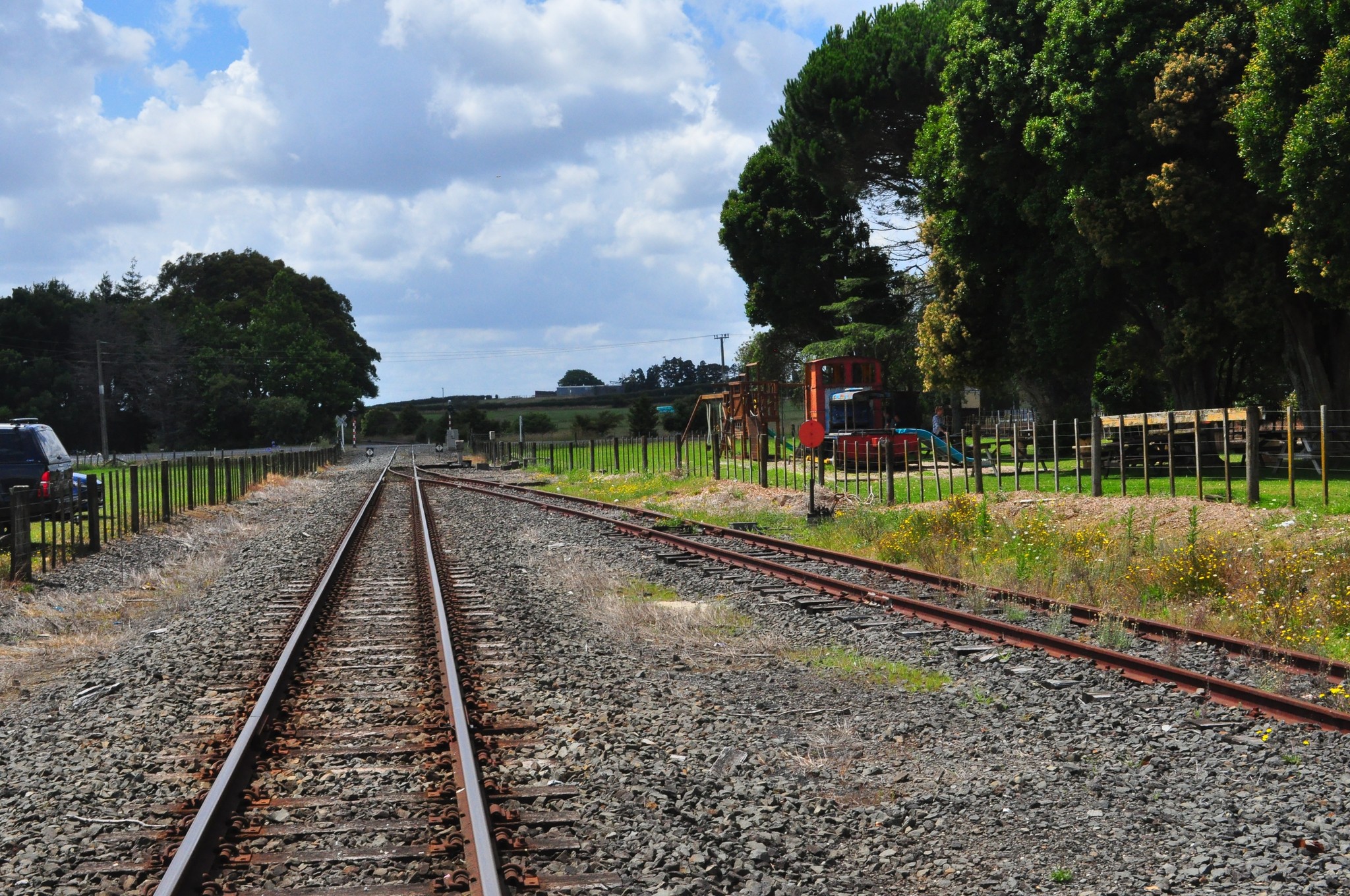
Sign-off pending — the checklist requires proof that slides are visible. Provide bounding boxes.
[894,428,991,467]
[757,423,797,452]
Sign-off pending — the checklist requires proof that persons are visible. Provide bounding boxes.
[893,415,903,428]
[272,441,276,447]
[933,406,949,437]
[884,413,892,428]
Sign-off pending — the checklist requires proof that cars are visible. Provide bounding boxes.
[48,473,104,520]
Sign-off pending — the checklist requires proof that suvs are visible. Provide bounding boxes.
[0,418,78,534]
